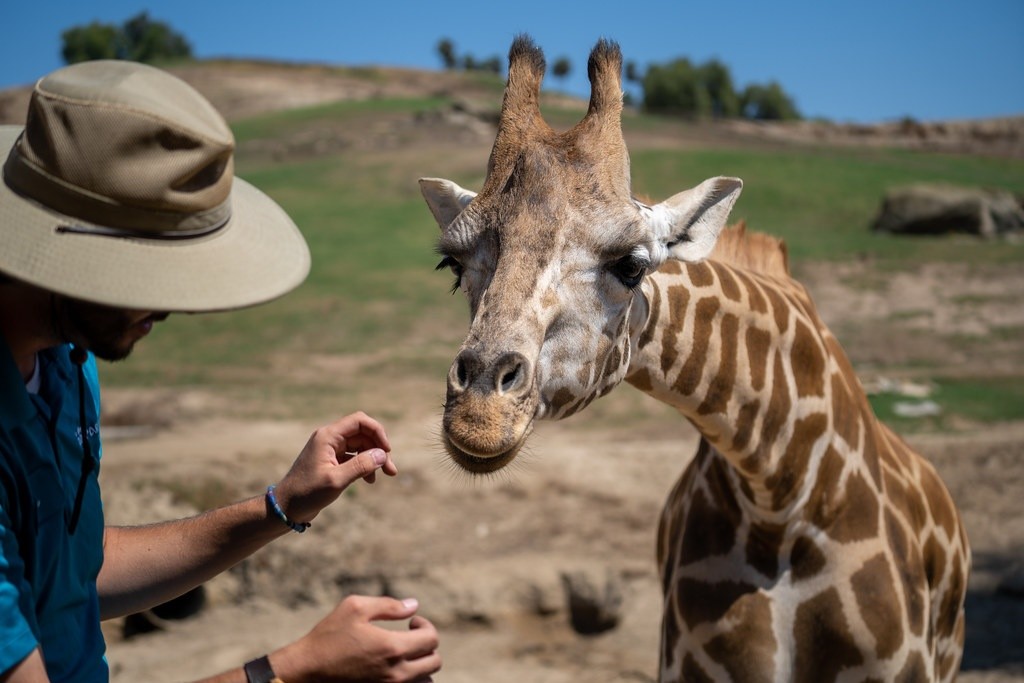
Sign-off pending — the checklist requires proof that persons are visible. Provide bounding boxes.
[0,60,446,683]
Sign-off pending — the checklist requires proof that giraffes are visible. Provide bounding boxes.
[415,37,972,683]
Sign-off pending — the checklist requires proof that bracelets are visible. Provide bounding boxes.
[268,481,312,533]
[244,653,285,683]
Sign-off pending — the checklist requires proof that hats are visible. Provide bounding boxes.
[0,59,313,311]
